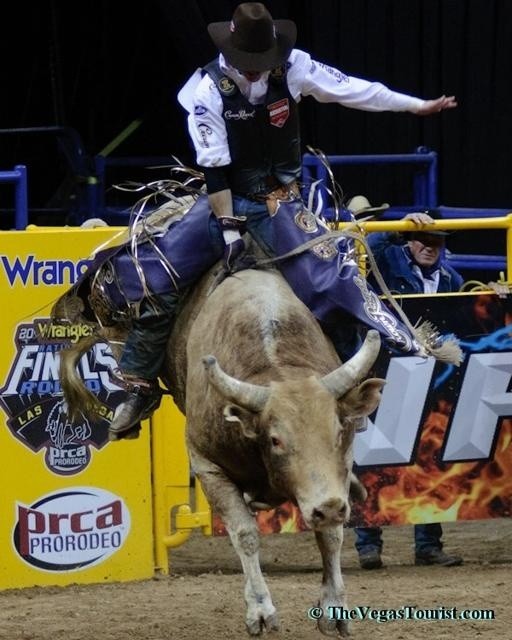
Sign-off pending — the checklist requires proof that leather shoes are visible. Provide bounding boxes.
[358,547,384,568]
[413,546,465,568]
[107,383,164,440]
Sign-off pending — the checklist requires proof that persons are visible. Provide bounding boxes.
[105,1,462,433]
[350,209,469,570]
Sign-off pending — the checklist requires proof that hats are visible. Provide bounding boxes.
[398,208,456,238]
[206,2,297,74]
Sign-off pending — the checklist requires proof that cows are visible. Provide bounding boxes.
[59,233,386,640]
